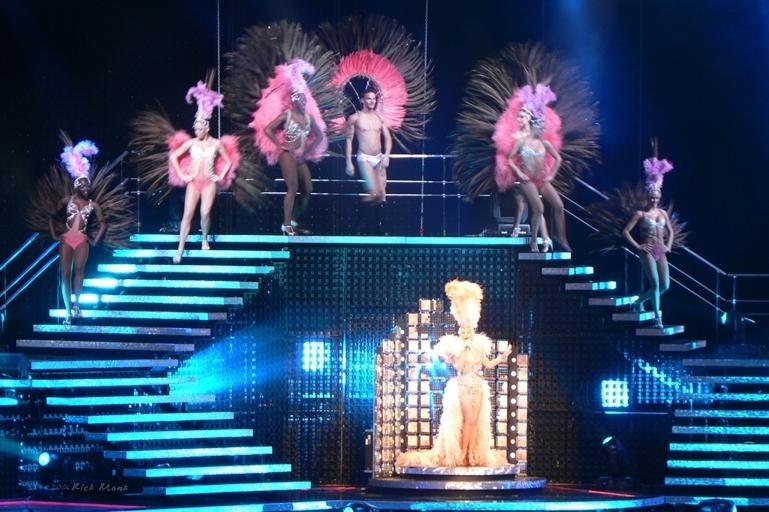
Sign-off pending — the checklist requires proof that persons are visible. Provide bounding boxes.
[507,118,578,254]
[47,174,107,328]
[624,185,675,330]
[510,108,550,253]
[344,90,390,236]
[264,92,324,237]
[170,114,234,265]
[447,323,513,469]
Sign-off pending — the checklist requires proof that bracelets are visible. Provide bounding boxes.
[501,355,508,361]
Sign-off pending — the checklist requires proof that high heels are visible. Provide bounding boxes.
[652,312,666,330]
[510,224,573,254]
[280,222,298,236]
[631,294,647,314]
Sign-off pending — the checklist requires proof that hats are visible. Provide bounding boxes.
[518,80,558,127]
[56,139,105,186]
[273,59,314,96]
[442,273,488,329]
[181,80,227,123]
[641,155,676,194]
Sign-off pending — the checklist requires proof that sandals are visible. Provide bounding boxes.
[200,236,214,252]
[172,247,185,265]
[60,305,82,324]
